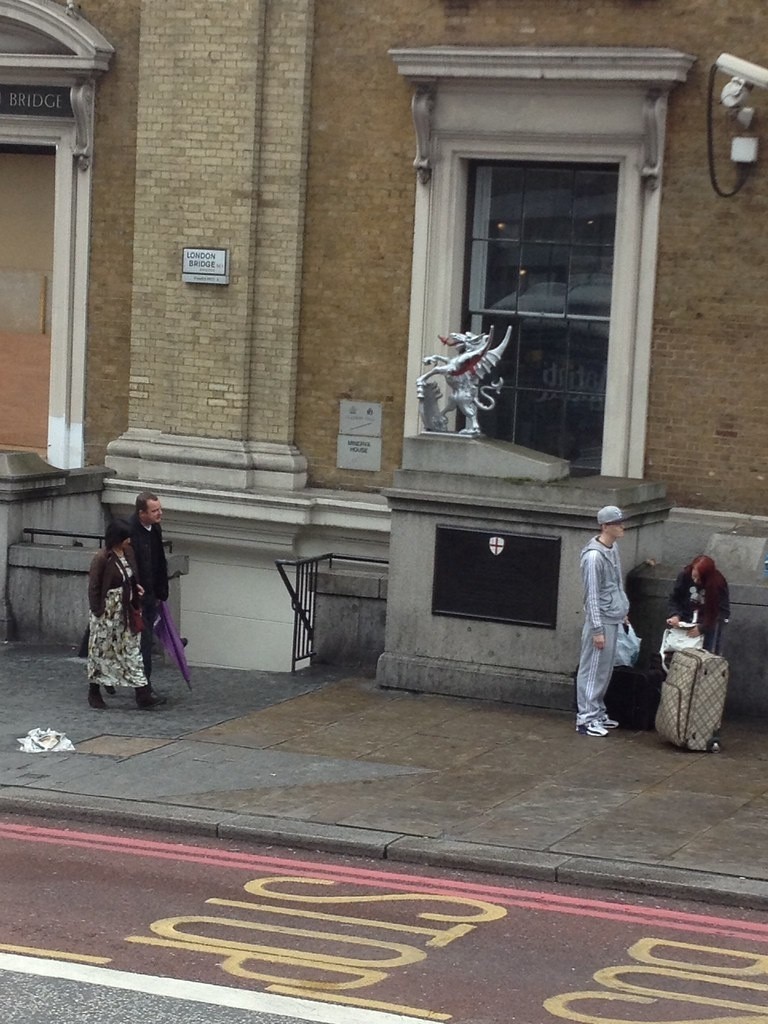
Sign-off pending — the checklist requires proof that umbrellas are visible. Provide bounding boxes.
[152,597,196,695]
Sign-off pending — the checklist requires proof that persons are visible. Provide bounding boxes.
[575,505,631,736]
[86,521,167,711]
[669,554,732,656]
[101,491,167,678]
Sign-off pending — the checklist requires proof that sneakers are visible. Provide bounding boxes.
[88,682,105,707]
[575,718,610,737]
[599,712,619,729]
[103,683,118,694]
[136,684,167,710]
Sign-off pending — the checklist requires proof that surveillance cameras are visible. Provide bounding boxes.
[717,52,768,108]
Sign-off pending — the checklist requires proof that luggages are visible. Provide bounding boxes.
[655,615,731,753]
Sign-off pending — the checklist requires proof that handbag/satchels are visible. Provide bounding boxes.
[658,621,705,672]
[614,618,642,667]
[127,602,145,634]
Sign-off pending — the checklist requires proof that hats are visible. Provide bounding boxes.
[597,505,625,525]
